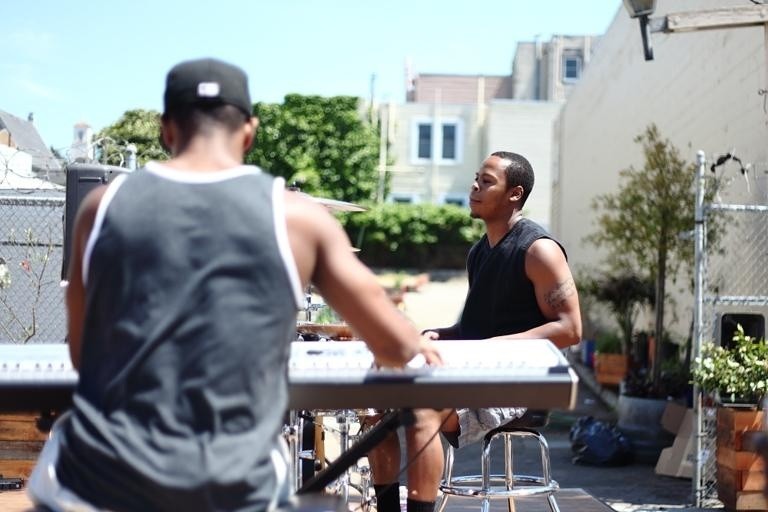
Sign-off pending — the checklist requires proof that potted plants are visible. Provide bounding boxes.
[689,321,765,512]
[616,382,665,437]
[594,335,629,385]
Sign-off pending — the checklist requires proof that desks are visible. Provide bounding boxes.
[1,337,579,510]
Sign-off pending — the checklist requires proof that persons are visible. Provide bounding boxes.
[360,151,582,512]
[26,55,445,512]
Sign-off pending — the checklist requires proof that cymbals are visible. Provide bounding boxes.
[290,186,371,212]
[296,324,355,340]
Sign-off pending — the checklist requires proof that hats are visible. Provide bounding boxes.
[159,58,253,153]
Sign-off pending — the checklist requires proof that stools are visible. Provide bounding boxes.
[440,399,564,511]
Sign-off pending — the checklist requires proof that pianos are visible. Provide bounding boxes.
[1,339,579,412]
